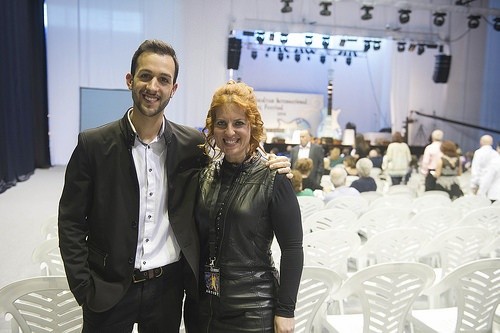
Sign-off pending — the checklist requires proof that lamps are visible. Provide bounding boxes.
[280,0,293,13]
[361,6,373,20]
[252,32,424,65]
[432,12,446,26]
[492,18,500,31]
[399,9,411,23]
[320,2,332,15]
[466,14,481,28]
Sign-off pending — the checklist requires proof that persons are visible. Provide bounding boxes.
[290,131,324,191]
[386,132,412,184]
[58,39,293,333]
[422,131,472,200]
[351,134,368,161]
[471,135,500,203]
[324,147,383,203]
[184,84,303,333]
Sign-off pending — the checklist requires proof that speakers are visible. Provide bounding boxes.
[228,37,241,71]
[433,55,452,82]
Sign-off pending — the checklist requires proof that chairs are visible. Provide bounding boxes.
[0,216,84,333]
[270,173,500,333]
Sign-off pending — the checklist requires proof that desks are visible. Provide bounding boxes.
[265,143,426,155]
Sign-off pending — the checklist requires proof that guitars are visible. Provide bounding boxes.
[315,79,342,142]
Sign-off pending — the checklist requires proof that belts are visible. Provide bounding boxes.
[131,259,185,284]
